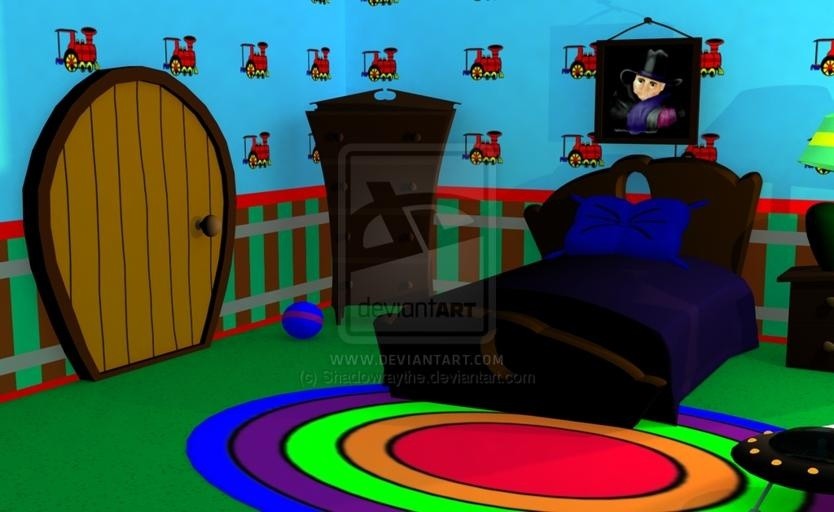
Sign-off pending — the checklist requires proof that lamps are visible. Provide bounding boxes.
[797,112,834,266]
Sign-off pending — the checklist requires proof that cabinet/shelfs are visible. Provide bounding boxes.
[304,87,462,324]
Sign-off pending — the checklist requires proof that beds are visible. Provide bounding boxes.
[374,153,764,432]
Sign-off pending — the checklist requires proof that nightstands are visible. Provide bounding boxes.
[775,265,833,371]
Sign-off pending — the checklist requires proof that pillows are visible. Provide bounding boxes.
[546,191,705,271]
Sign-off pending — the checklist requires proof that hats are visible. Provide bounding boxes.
[620,49,684,88]
